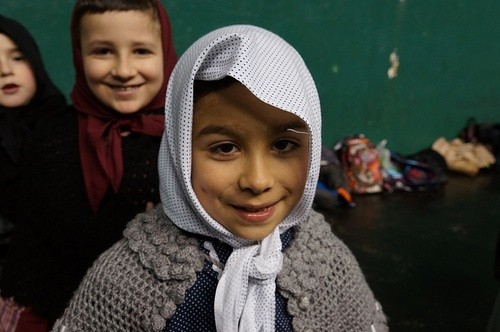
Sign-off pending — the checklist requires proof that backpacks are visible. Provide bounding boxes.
[337,133,386,195]
[311,145,356,218]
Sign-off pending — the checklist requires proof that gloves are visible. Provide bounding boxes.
[434,135,494,174]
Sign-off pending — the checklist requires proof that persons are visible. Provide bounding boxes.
[17,0,178,332]
[0,16,69,332]
[52,24,389,332]
